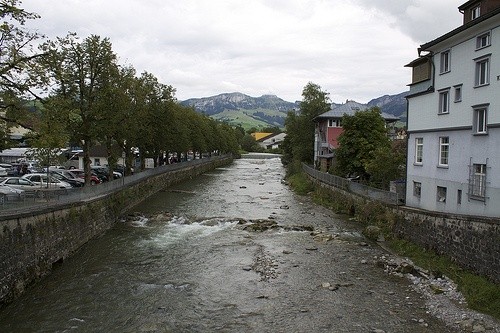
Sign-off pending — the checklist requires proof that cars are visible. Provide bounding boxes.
[0,148,207,205]
